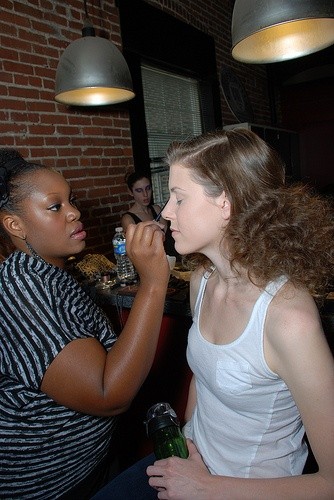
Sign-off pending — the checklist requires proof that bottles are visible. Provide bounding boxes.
[112,227,134,279]
[142,403,189,461]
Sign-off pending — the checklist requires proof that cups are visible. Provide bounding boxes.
[101,271,110,282]
[166,255,176,270]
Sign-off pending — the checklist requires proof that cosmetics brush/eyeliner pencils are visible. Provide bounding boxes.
[154,196,170,222]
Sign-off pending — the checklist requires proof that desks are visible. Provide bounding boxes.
[80,269,334,365]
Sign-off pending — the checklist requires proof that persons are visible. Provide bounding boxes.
[120,171,175,258]
[0,147,170,500]
[91,128,334,500]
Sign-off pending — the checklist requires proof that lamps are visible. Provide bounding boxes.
[54,0,135,107]
[229,0,334,65]
